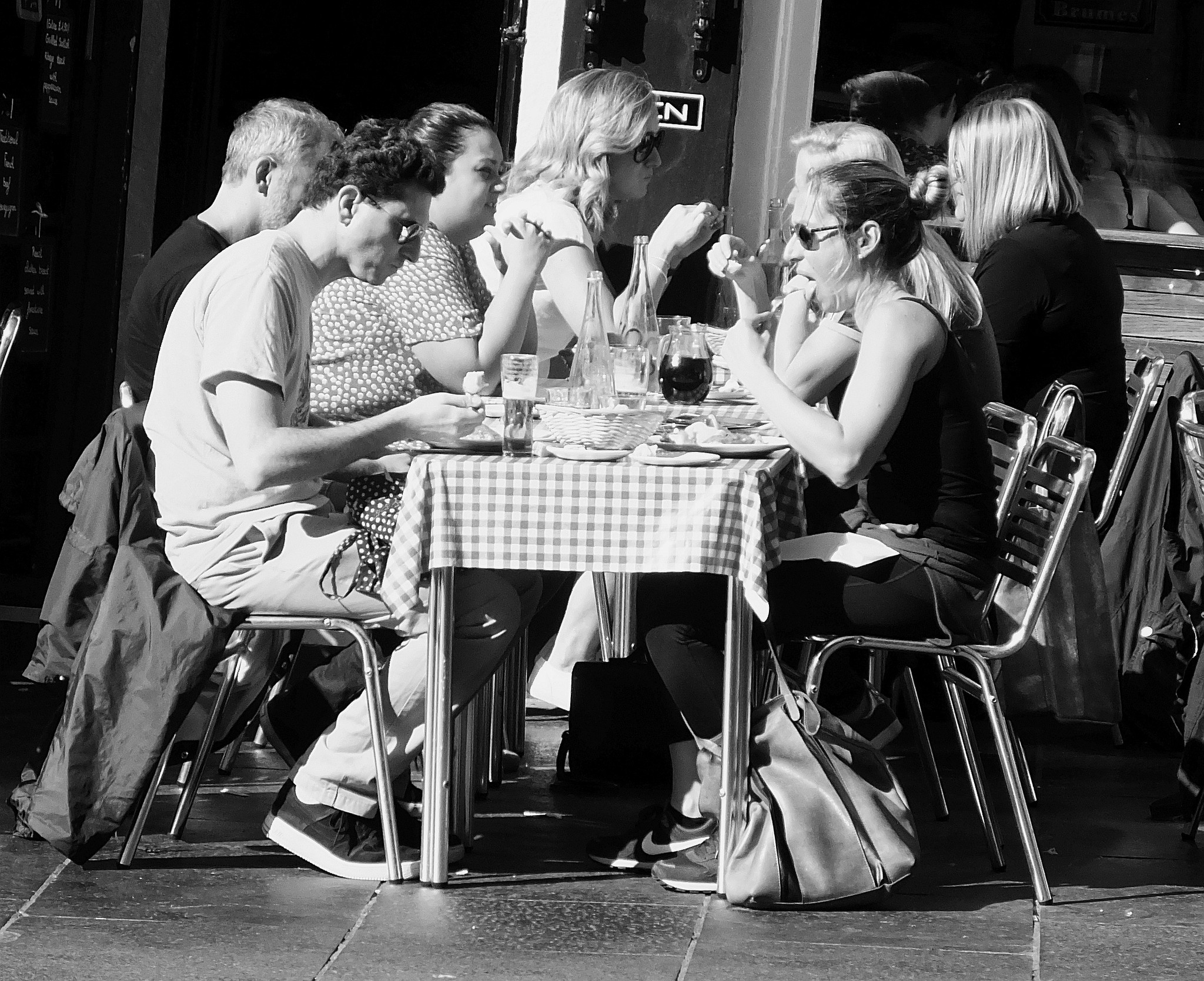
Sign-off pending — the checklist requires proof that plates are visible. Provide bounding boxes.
[631,451,720,465]
[649,433,792,457]
[547,444,630,461]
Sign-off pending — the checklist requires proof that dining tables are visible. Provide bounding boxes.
[375,350,831,890]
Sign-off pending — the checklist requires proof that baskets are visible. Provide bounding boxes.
[534,405,666,449]
[696,323,728,355]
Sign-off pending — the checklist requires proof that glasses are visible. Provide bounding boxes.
[362,191,426,244]
[789,223,862,250]
[615,128,666,163]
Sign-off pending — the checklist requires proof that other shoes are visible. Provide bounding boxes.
[501,747,520,770]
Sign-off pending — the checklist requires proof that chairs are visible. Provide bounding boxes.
[735,345,1204,904]
[119,382,529,886]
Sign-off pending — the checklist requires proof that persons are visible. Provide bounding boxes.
[1060,96,1199,239]
[946,99,1129,519]
[123,104,550,882]
[469,68,723,362]
[837,69,948,177]
[592,120,996,894]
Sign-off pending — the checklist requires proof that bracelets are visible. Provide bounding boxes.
[649,264,668,283]
[649,255,670,270]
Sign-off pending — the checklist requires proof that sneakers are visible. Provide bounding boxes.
[393,801,465,862]
[261,778,423,880]
[653,832,718,894]
[592,818,717,870]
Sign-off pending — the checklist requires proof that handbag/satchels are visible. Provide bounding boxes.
[568,648,693,786]
[698,637,917,907]
[319,472,403,599]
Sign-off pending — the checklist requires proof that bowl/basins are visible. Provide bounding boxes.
[427,397,533,451]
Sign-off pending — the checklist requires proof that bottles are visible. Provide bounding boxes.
[714,206,741,328]
[756,198,788,298]
[618,236,660,393]
[569,270,617,408]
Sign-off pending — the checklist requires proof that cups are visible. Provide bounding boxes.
[545,387,571,408]
[659,326,713,406]
[658,315,691,360]
[609,344,649,410]
[500,354,539,456]
[707,388,750,399]
